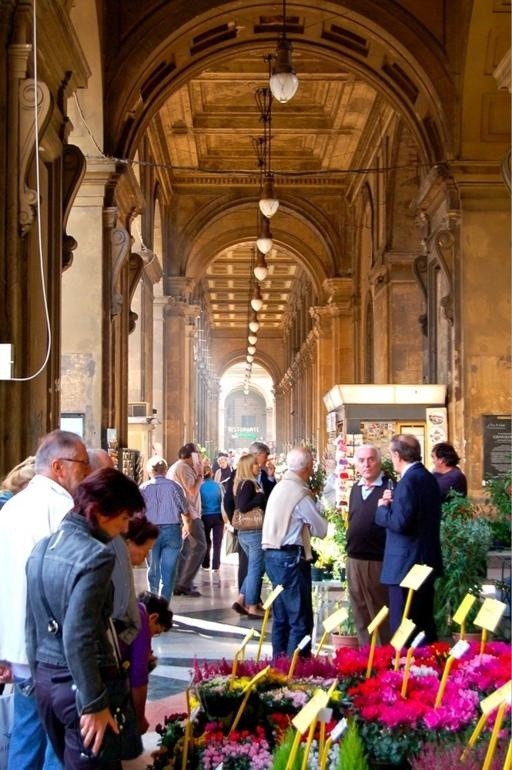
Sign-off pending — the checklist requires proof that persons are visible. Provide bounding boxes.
[1,427,468,770]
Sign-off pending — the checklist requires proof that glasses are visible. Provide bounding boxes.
[60,458,88,466]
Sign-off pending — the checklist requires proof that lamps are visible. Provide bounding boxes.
[244,0,298,395]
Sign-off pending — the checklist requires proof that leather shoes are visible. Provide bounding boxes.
[232,602,272,619]
[174,586,200,597]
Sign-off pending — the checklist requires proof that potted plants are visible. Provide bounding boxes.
[324,507,359,651]
[434,485,496,645]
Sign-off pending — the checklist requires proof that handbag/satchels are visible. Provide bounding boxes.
[77,671,142,762]
[232,507,264,529]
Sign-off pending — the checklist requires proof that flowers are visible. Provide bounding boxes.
[145,640,512,770]
